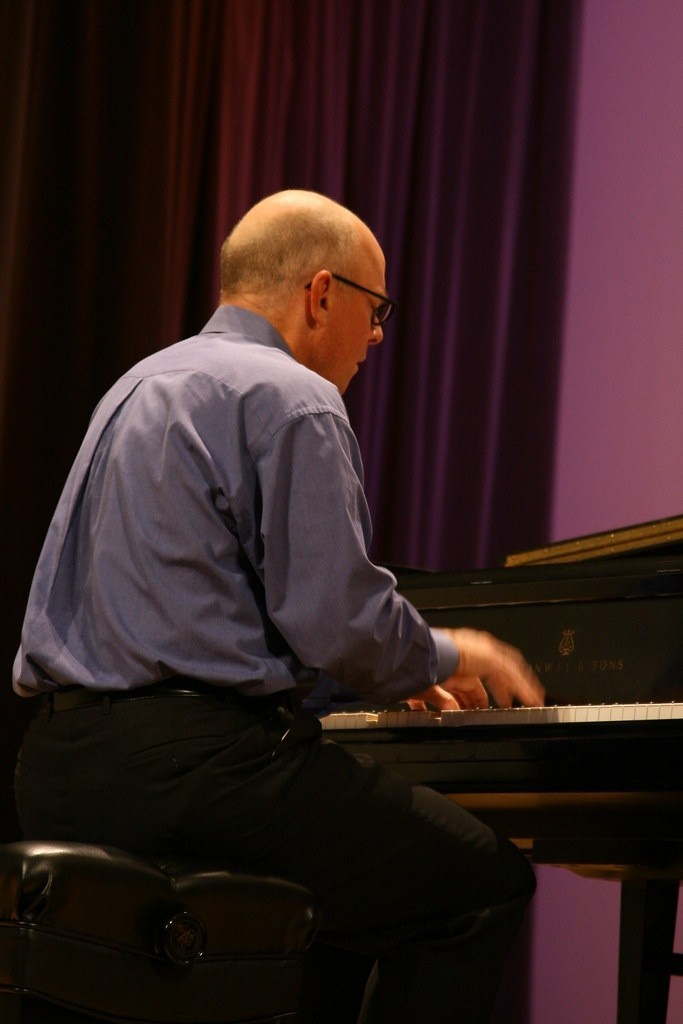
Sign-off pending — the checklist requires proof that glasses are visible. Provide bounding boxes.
[305,274,395,325]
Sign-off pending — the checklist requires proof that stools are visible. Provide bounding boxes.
[0,838,324,1024]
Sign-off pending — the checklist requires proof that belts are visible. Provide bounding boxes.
[37,681,246,716]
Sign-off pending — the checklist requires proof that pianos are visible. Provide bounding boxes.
[319,511,683,1024]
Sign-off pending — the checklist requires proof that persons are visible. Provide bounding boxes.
[10,189,545,1024]
